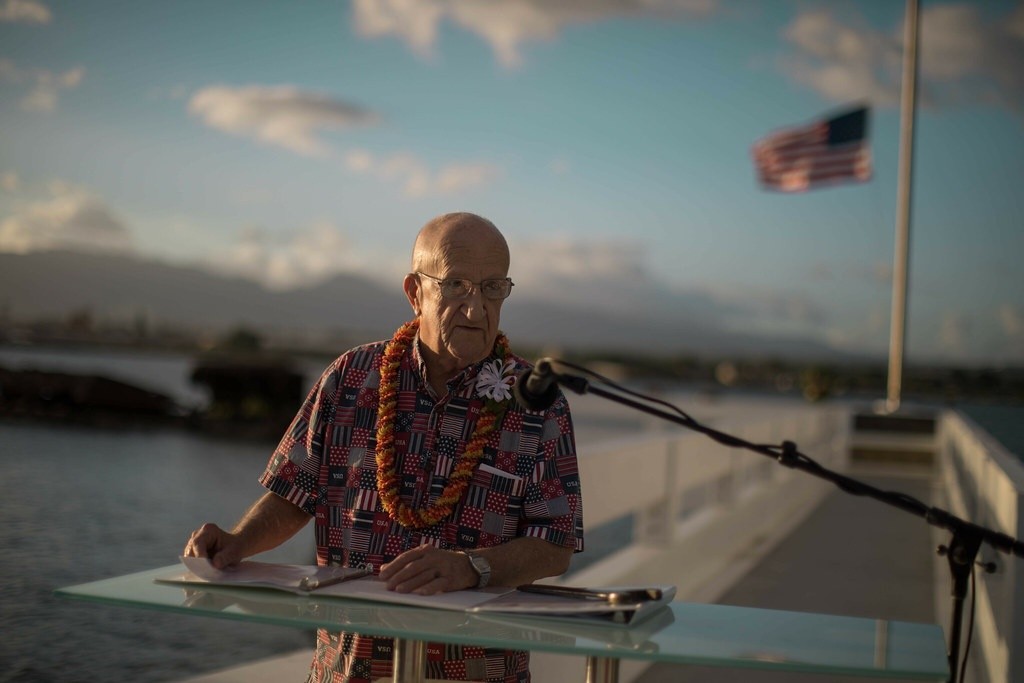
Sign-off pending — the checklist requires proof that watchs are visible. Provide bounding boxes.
[467,551,491,588]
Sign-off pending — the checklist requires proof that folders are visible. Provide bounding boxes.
[156,558,516,612]
[466,585,677,631]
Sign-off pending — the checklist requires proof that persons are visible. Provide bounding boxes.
[185,212,585,683]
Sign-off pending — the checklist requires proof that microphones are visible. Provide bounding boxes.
[512,358,560,412]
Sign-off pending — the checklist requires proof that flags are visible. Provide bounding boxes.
[750,101,872,194]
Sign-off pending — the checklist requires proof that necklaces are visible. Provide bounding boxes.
[375,316,515,530]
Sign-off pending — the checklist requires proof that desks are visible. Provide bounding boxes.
[51,561,951,683]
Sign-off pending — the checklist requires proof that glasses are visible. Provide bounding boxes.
[414,271,515,301]
